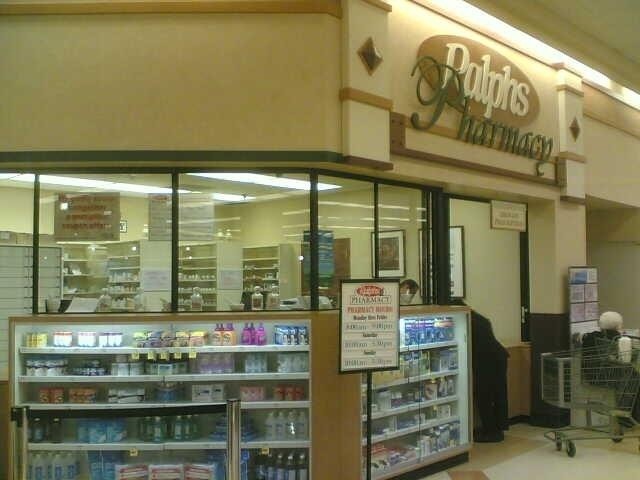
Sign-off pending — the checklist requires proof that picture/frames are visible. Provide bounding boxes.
[371,225,466,299]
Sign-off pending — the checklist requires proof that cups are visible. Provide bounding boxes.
[46,298,59,313]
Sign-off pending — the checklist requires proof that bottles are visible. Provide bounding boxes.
[191,288,201,309]
[252,286,279,311]
[29,412,308,480]
[98,289,111,311]
[214,322,265,344]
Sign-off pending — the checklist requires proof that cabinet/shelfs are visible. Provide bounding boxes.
[8,316,311,478]
[359,308,473,479]
[63,239,299,312]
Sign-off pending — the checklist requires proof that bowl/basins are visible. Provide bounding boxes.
[60,300,72,312]
[229,304,245,310]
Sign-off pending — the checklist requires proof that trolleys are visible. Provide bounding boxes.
[540,336,639,457]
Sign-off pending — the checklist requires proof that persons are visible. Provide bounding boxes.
[582,327,640,427]
[400,279,418,305]
[452,298,509,443]
